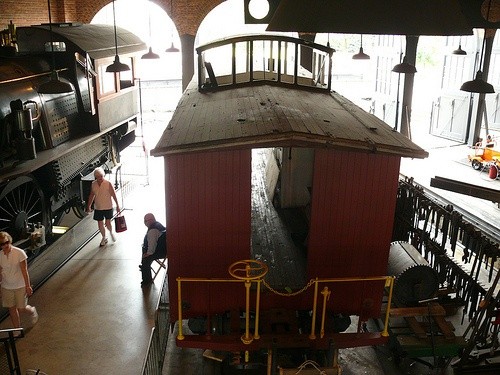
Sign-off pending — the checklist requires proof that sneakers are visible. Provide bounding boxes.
[100,239,108,245]
[13,331,21,340]
[31,306,38,324]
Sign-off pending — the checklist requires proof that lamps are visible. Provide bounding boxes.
[140,15,160,59]
[105,0,130,73]
[325,33,333,58]
[352,33,371,60]
[36,0,75,94]
[391,35,418,74]
[452,36,467,55]
[459,0,496,93]
[165,33,179,53]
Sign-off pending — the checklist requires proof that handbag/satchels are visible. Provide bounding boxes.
[114,211,127,232]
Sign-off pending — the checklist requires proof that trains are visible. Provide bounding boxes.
[0,21,149,275]
[150,36,429,375]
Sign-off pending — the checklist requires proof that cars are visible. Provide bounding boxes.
[467,139,500,178]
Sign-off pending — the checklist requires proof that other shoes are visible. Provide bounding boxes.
[139,265,143,268]
[141,280,153,284]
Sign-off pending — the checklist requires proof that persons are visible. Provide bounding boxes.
[86,167,121,247]
[482,133,494,149]
[0,232,39,336]
[138,213,167,284]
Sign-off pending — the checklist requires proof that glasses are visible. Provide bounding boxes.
[0,241,10,246]
